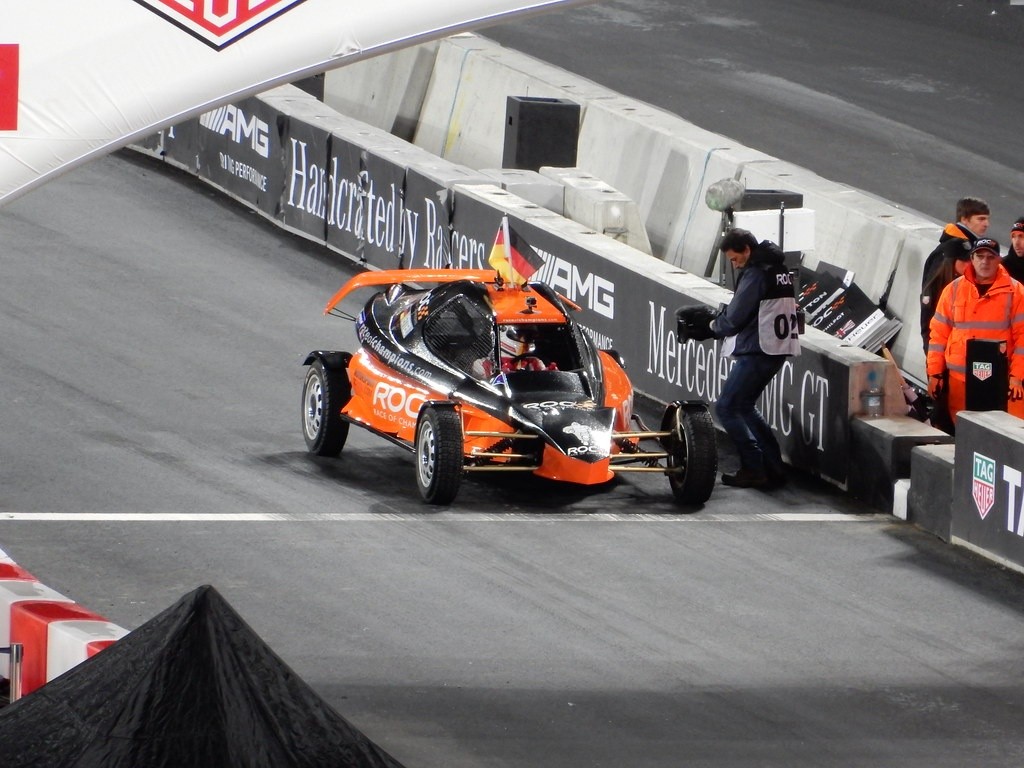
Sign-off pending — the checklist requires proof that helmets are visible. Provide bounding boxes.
[491,321,536,358]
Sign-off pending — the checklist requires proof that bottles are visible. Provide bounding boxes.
[860,371,882,416]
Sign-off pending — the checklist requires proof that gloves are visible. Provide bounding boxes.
[1008,376,1024,402]
[928,372,944,399]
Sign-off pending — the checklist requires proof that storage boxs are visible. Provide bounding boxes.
[732,208,816,254]
[480,168,564,215]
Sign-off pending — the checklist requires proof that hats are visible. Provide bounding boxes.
[970,238,1000,256]
[1009,217,1024,237]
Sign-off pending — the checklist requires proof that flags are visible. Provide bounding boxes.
[488,221,545,286]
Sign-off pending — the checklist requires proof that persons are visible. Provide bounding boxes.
[1002,216,1024,285]
[472,325,559,382]
[675,228,800,491]
[921,197,991,354]
[926,238,1024,429]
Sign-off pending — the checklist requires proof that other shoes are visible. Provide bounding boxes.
[721,458,773,493]
[762,460,787,485]
